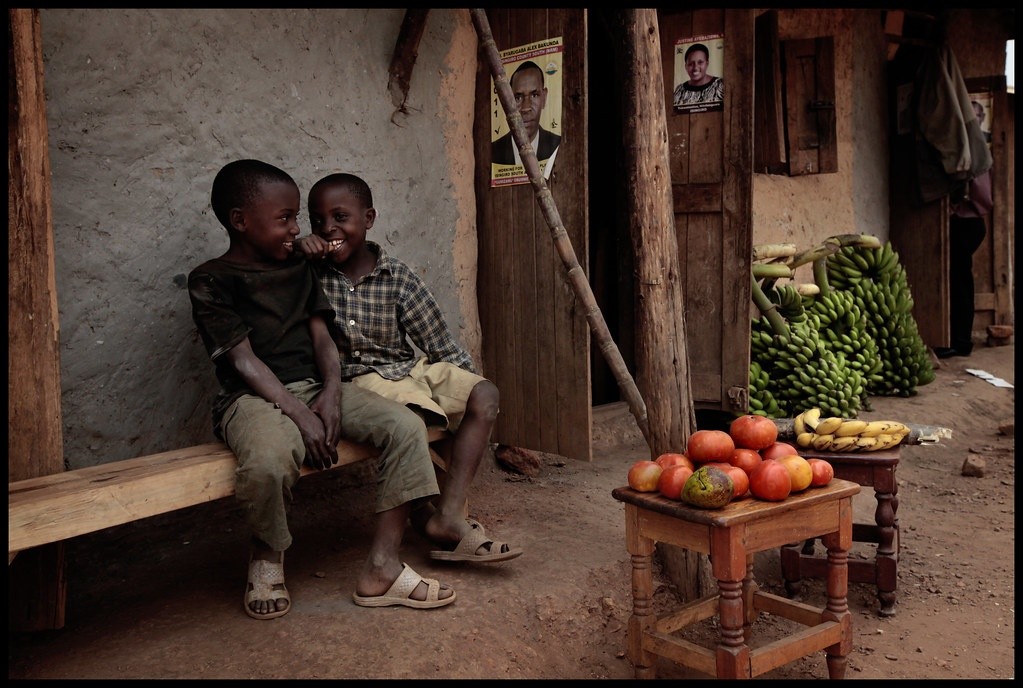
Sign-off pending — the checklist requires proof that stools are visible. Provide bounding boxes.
[612,478,861,679]
[776,436,901,618]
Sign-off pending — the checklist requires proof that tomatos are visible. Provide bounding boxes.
[628,414,834,501]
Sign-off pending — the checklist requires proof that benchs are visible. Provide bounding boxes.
[9,425,468,634]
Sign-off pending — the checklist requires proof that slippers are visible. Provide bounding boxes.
[244,548,290,620]
[465,518,485,535]
[431,530,523,562]
[353,563,456,608]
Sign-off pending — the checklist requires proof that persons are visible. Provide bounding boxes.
[972,101,992,143]
[932,169,994,360]
[187,160,456,619]
[491,62,561,165]
[293,173,523,563]
[673,44,723,105]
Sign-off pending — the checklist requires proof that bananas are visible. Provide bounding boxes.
[728,239,938,423]
[793,407,911,452]
